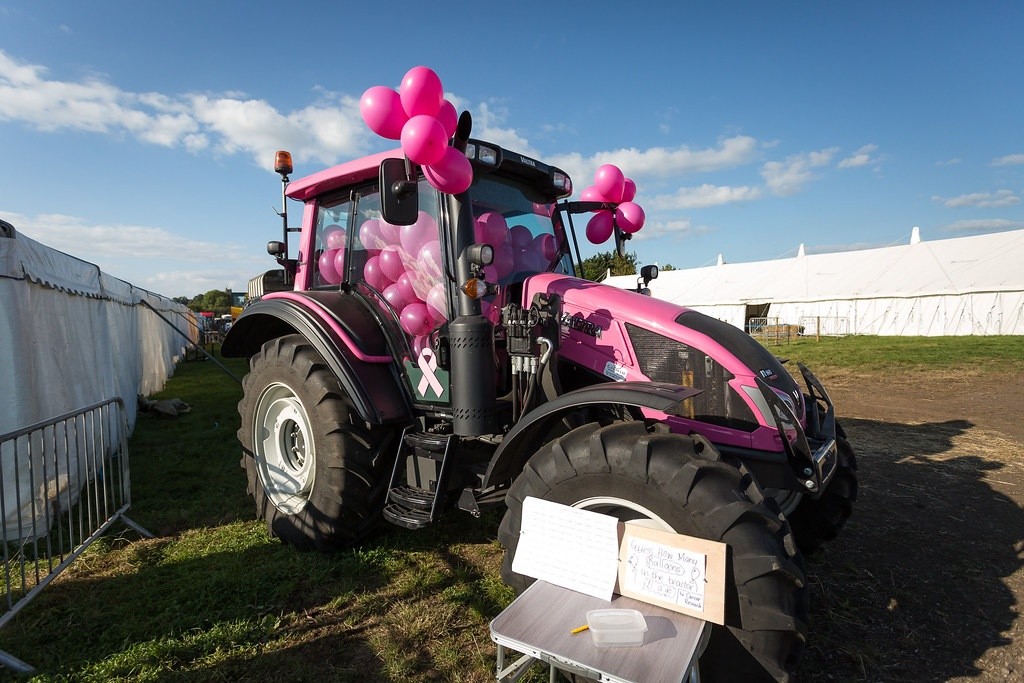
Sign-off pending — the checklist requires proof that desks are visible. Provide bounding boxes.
[489,578,706,683]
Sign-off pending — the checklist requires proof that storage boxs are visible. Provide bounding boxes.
[586,609,648,647]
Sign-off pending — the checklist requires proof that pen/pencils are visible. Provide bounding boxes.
[571,624,590,634]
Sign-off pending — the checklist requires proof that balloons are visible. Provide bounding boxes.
[596,164,623,204]
[360,85,410,139]
[619,177,636,203]
[319,210,563,364]
[585,211,613,244]
[435,100,457,139]
[420,145,473,193]
[400,115,447,165]
[402,66,442,118]
[582,188,610,212]
[616,203,645,232]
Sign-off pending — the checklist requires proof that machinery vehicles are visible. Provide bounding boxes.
[221,109,859,683]
